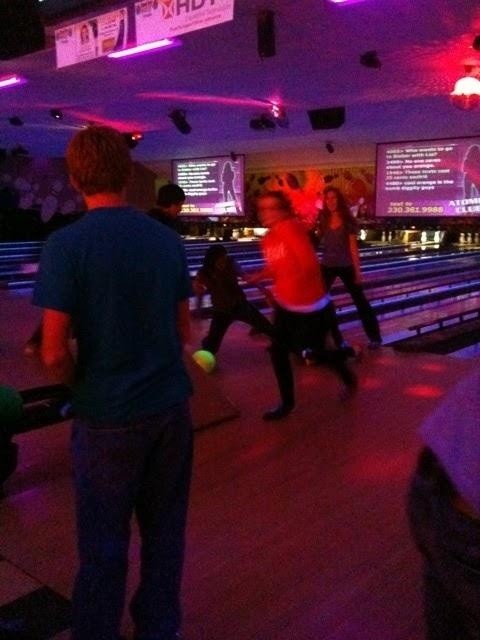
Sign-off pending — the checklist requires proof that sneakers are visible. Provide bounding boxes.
[342,341,380,356]
[340,378,356,400]
[263,402,292,421]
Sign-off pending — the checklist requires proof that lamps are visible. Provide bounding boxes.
[164,107,192,139]
[246,113,274,132]
[446,57,479,100]
[252,9,276,58]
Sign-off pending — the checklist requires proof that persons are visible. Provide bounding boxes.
[36,126,195,640]
[193,244,274,353]
[405,367,479,639]
[146,184,187,235]
[308,185,383,354]
[240,188,357,418]
